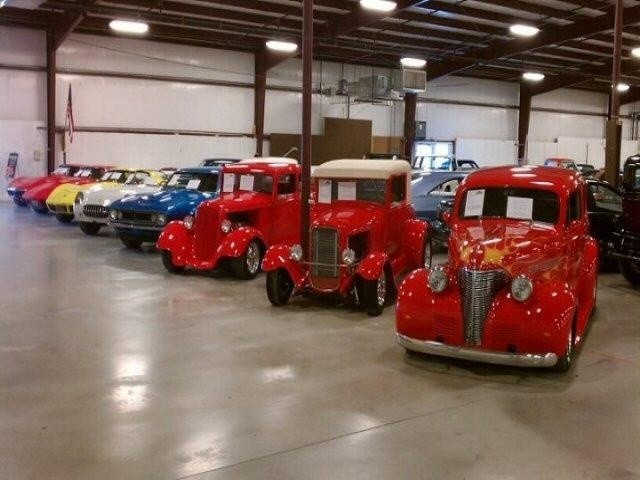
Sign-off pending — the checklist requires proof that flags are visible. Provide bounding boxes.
[66,85,75,144]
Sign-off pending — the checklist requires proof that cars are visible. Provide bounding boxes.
[5,163,170,237]
[408,156,639,295]
[392,163,600,374]
[264,158,434,316]
[155,156,302,279]
[105,166,221,250]
